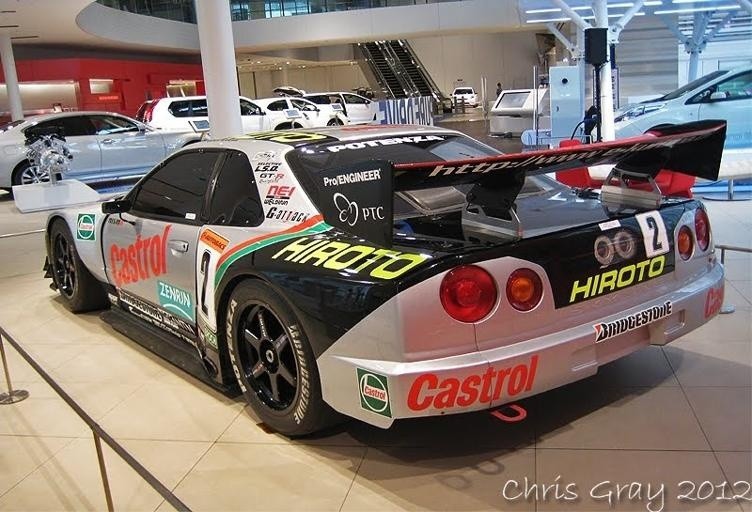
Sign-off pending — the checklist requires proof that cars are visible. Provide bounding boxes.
[449,87,479,108]
[614,64,751,153]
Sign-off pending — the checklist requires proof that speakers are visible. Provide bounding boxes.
[585,27,608,67]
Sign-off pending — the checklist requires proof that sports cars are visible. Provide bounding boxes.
[39,121,730,439]
